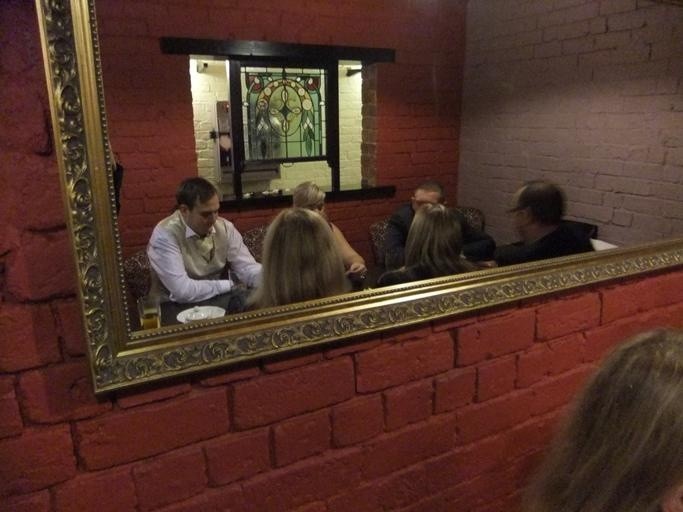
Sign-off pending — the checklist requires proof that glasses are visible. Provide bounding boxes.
[505,207,521,218]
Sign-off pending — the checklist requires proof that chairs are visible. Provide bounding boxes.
[122,224,267,304]
[368,206,485,270]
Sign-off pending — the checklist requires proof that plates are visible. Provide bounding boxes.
[176,306,225,324]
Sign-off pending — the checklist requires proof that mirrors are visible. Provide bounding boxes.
[37,0,683,397]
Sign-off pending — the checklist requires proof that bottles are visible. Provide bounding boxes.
[135,293,161,330]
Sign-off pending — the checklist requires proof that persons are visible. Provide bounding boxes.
[509,325,683,510]
[287,180,371,281]
[144,175,263,307]
[385,179,498,263]
[491,178,598,268]
[373,202,476,289]
[235,205,353,313]
